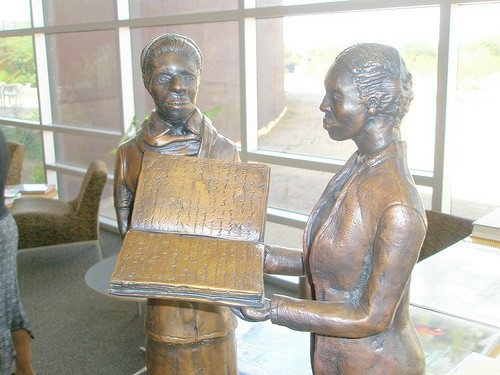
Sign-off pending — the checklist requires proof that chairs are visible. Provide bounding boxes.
[11,160,108,261]
[6,141,25,185]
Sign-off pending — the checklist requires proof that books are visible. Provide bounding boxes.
[111,151,274,312]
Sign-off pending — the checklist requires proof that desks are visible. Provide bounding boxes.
[4,183,58,199]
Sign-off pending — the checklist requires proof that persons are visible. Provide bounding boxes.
[234,43,428,375]
[111,30,254,375]
[0,129,42,375]
